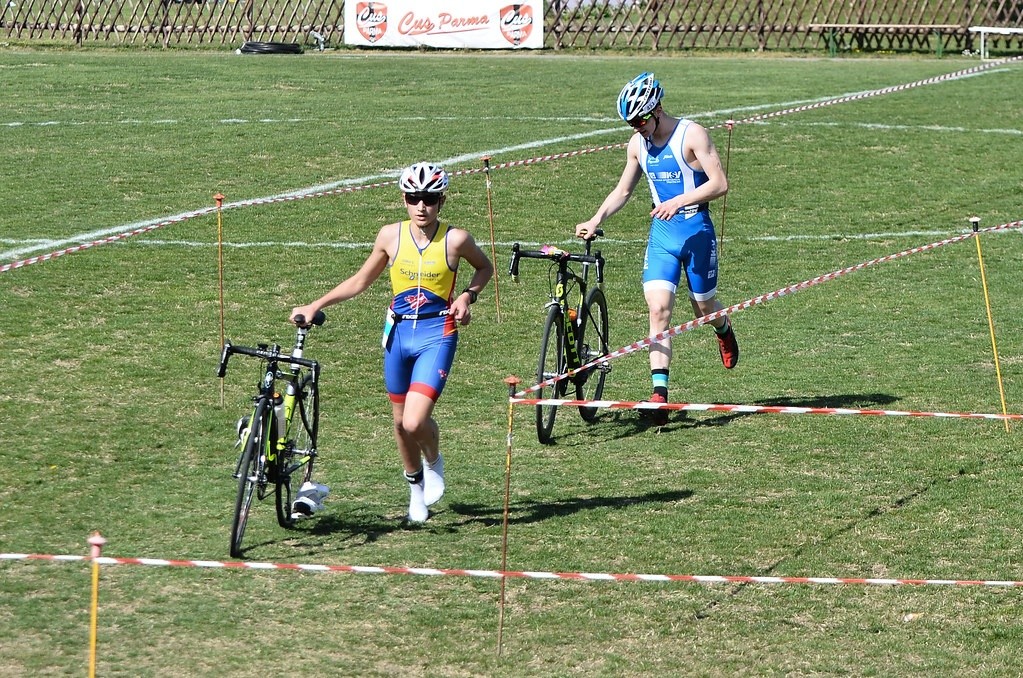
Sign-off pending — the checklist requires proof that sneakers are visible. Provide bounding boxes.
[638,393,669,425]
[403,466,429,522]
[423,450,445,506]
[714,318,739,369]
[294,481,328,516]
[235,418,255,460]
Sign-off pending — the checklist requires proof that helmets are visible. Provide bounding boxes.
[399,161,449,193]
[616,72,665,121]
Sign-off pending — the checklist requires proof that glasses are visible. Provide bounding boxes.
[405,193,439,206]
[628,113,652,128]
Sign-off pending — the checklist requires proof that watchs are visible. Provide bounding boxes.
[462,289,477,304]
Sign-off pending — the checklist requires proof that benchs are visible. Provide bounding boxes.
[807,24,961,60]
[969,27,1023,60]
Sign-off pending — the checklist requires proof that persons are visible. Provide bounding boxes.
[575,72,738,425]
[289,161,494,522]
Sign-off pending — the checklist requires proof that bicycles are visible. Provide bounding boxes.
[507,228,613,444]
[218,311,327,557]
